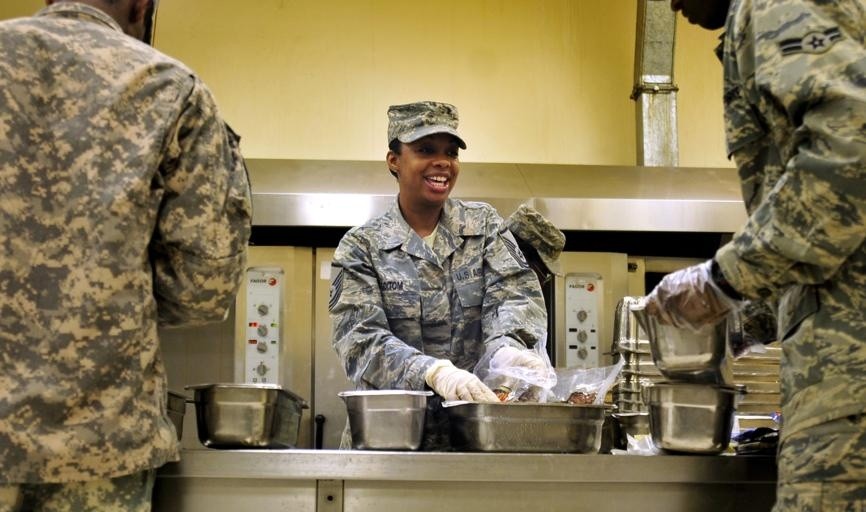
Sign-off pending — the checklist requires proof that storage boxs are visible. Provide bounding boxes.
[641,381,746,454]
[443,399,618,453]
[338,389,433,451]
[166,390,187,439]
[630,303,722,381]
[185,383,309,447]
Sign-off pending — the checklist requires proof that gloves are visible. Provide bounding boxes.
[424,347,550,404]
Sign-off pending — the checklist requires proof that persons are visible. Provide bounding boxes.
[0,2,255,512]
[640,0,866,512]
[327,102,551,451]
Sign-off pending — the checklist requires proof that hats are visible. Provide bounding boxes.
[387,100,468,150]
[504,202,567,279]
